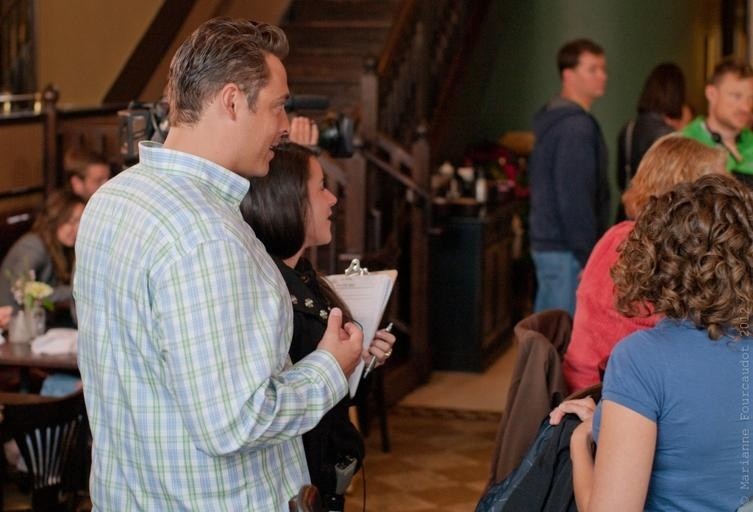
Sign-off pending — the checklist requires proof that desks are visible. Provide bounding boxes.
[0,341,78,396]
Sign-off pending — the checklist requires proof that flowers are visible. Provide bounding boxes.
[1,248,57,313]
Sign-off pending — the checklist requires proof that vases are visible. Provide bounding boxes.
[26,305,47,336]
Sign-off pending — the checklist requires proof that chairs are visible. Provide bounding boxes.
[280,482,323,511]
[511,301,573,379]
[0,383,91,512]
[554,381,602,411]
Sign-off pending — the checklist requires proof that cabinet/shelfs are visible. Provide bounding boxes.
[427,191,530,377]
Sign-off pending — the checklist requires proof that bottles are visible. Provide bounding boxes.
[8,308,29,342]
[474,168,486,202]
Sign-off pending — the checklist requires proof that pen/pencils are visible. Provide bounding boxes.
[363,322,394,379]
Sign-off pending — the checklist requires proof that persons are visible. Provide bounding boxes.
[0,16,752,512]
